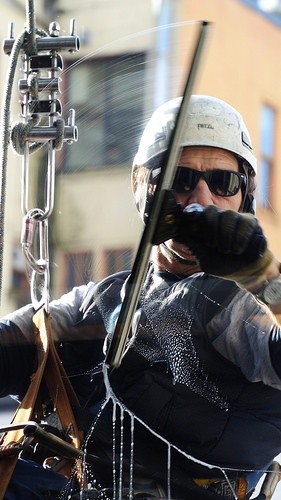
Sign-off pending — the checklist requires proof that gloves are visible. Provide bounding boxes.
[169,202,274,289]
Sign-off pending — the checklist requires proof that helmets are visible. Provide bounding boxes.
[134,94,258,178]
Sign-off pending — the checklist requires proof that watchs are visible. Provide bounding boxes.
[252,274,281,305]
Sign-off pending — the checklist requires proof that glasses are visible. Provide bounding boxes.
[151,165,246,197]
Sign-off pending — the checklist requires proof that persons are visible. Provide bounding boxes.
[0,96,281,500]
